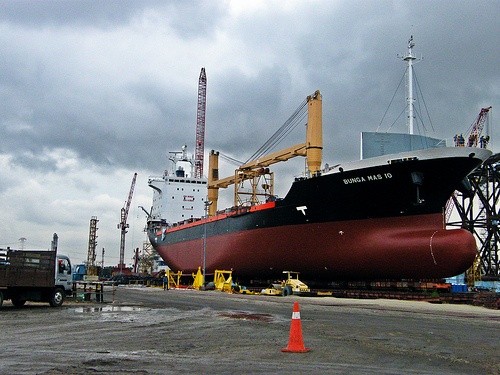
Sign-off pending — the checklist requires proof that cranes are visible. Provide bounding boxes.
[111,173,138,278]
[192,67,206,181]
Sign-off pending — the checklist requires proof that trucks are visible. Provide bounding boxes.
[0,250,74,308]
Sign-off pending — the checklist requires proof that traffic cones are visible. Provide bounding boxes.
[280,300,312,352]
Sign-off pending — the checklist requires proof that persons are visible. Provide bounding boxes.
[163,274,169,290]
[454,133,491,148]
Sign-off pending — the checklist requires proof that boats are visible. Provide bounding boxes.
[143,35,494,286]
